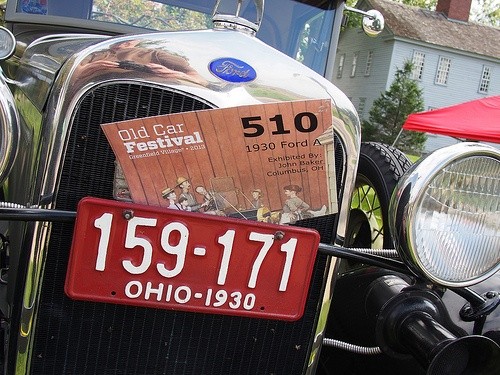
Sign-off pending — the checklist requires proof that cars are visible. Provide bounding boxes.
[0,0,500,375]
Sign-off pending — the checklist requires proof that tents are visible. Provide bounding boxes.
[389,94,500,148]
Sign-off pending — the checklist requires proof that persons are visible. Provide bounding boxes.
[70,37,209,90]
